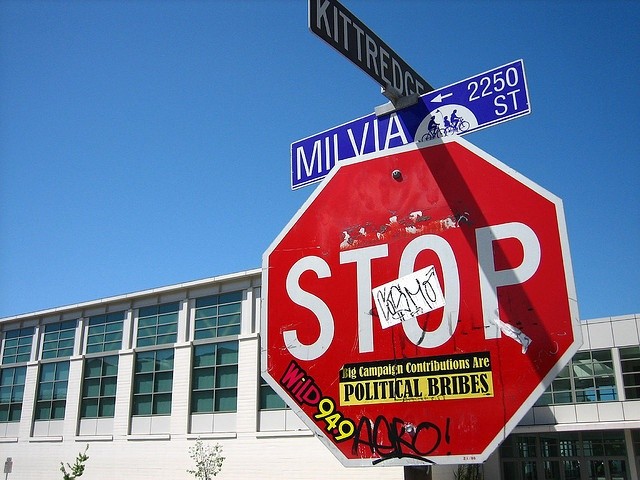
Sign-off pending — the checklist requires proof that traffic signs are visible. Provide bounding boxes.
[306,0,433,99]
[289,58,533,191]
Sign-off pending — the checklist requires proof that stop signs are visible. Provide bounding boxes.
[260,134,584,468]
